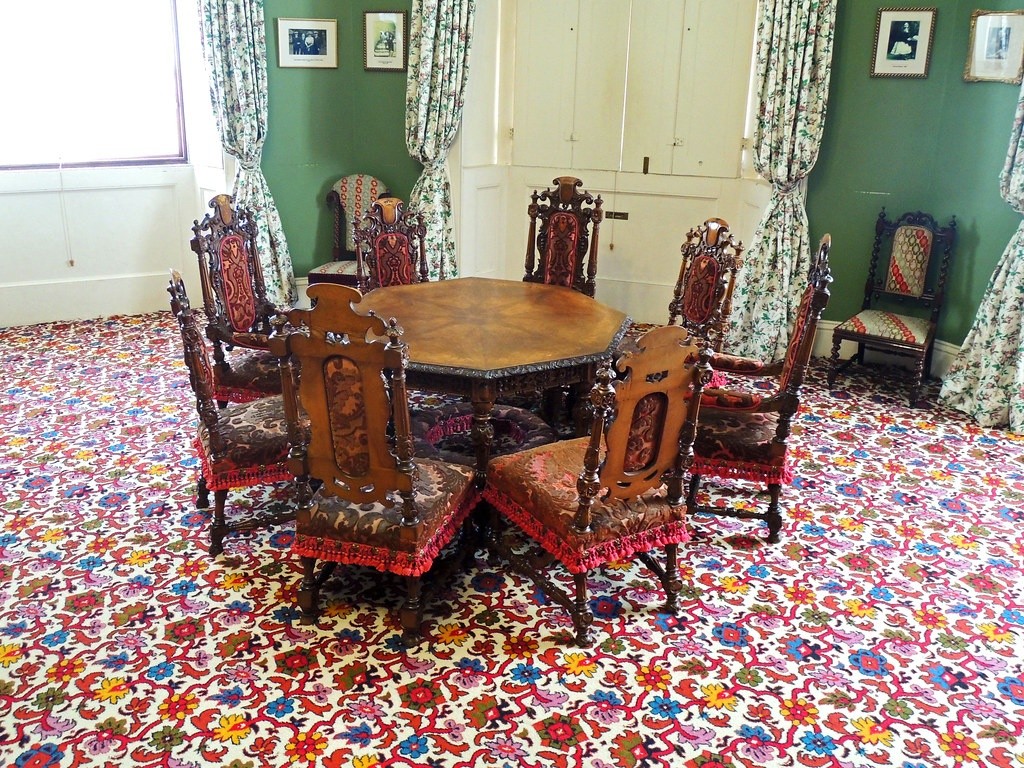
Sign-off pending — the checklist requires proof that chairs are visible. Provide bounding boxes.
[168,174,956,648]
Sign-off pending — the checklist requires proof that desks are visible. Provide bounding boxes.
[346,277,631,490]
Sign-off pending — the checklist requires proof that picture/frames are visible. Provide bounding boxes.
[964,9,1024,85]
[277,18,338,69]
[363,9,408,73]
[870,7,937,79]
